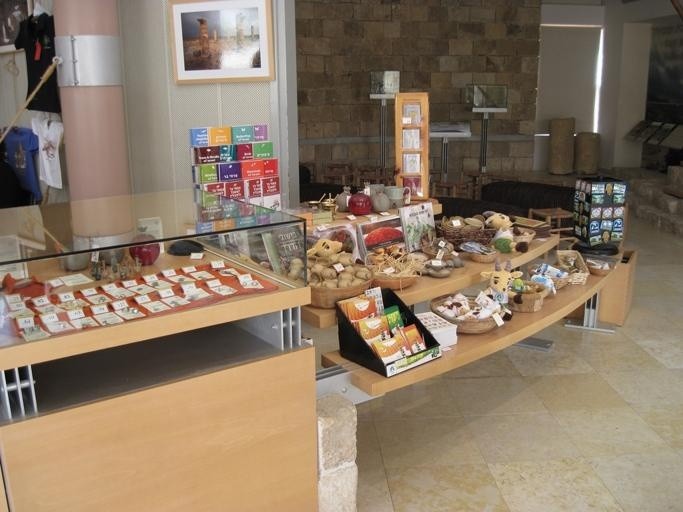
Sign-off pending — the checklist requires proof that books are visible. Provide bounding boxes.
[413,310,458,348]
[190,122,282,266]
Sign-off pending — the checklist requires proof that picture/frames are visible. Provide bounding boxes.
[167,0,275,85]
[1,1,34,55]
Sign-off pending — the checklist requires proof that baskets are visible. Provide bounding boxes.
[421,242,454,261]
[440,216,496,252]
[527,262,569,290]
[501,226,537,246]
[583,258,612,276]
[505,279,550,312]
[283,264,373,309]
[432,294,501,335]
[555,249,590,286]
[468,250,497,264]
[371,275,420,291]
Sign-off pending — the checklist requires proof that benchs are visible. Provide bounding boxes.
[433,180,628,232]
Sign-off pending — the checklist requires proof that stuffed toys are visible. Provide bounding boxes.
[479,256,523,319]
[482,210,529,252]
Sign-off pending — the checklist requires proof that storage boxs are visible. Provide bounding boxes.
[563,248,637,328]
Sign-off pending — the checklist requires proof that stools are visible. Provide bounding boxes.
[299,162,494,201]
[527,206,575,261]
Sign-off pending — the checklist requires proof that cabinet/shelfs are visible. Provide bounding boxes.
[293,198,624,400]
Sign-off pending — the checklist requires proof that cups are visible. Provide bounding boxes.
[308,199,338,221]
